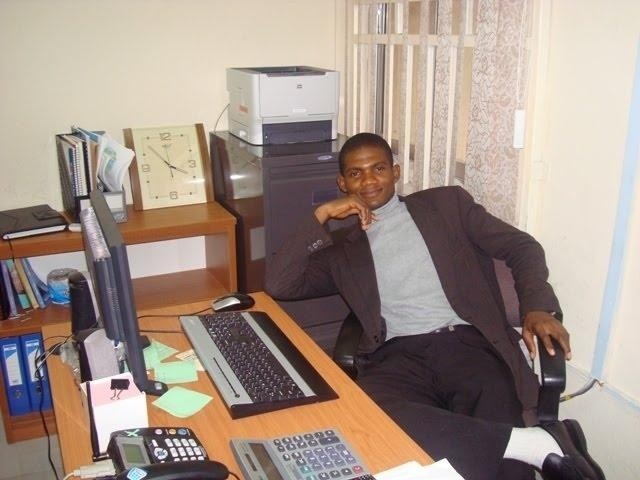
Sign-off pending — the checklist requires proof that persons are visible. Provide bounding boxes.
[263,132,605,480]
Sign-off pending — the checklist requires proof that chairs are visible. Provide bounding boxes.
[332,256,566,426]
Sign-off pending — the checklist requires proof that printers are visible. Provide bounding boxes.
[226,66,340,146]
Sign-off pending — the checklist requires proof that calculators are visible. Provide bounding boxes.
[231,427,371,480]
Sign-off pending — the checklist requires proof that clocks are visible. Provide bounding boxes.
[123,122,215,213]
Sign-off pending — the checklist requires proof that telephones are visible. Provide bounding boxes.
[107,427,228,480]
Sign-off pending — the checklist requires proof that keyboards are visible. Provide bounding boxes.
[179,312,320,420]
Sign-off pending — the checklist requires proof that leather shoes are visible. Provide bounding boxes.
[533,419,605,480]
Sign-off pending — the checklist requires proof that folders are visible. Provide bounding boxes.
[1,332,52,416]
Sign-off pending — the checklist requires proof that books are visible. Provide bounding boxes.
[1,257,51,321]
[56,125,101,197]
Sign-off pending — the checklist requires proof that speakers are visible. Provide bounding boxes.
[68,269,100,335]
[78,328,125,382]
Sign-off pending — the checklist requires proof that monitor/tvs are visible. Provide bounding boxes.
[77,188,168,396]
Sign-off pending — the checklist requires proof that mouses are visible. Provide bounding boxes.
[211,293,255,311]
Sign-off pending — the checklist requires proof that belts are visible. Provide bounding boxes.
[434,325,475,333]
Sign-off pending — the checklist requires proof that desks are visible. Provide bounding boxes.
[42,290,436,480]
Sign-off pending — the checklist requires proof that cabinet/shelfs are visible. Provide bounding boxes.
[209,130,354,358]
[1,201,238,446]
